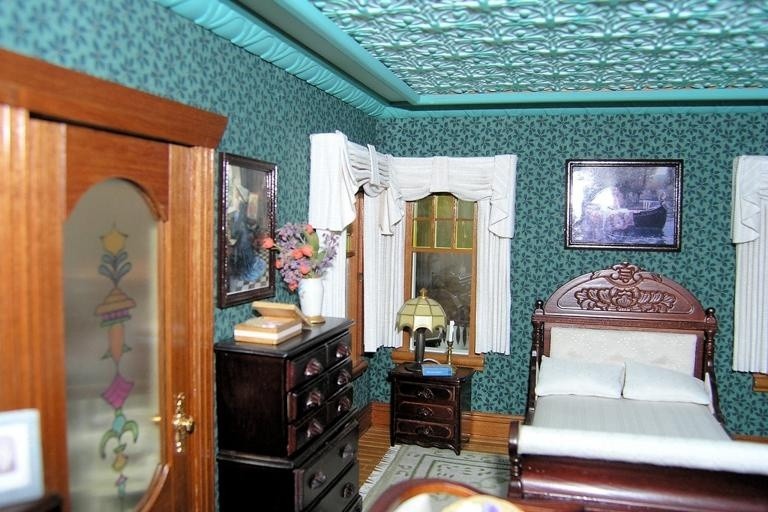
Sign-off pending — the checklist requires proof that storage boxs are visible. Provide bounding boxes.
[233,301,313,345]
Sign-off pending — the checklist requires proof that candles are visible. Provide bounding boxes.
[448,320,454,342]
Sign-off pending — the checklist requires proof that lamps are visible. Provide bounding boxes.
[395,288,447,372]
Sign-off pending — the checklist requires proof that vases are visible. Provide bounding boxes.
[298,278,325,324]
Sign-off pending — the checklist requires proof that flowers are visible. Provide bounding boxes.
[262,222,343,292]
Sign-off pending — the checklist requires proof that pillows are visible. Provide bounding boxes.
[535,327,711,405]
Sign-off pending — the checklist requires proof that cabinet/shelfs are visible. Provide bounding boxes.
[212,315,363,512]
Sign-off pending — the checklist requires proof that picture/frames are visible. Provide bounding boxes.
[565,159,683,253]
[217,151,277,310]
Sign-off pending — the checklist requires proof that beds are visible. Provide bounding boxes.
[508,261,768,512]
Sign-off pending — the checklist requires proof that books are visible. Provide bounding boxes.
[251,301,313,328]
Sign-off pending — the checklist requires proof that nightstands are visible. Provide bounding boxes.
[389,362,477,455]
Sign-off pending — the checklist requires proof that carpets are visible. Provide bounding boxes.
[358,444,512,512]
